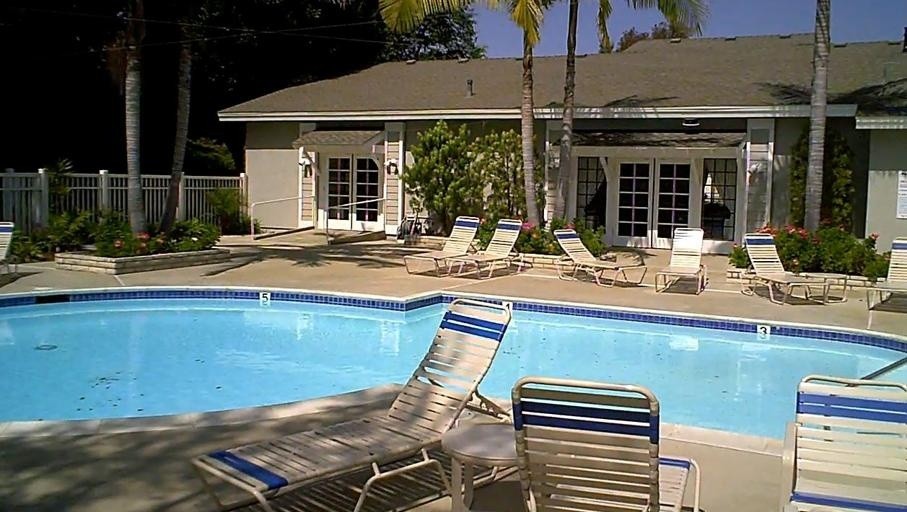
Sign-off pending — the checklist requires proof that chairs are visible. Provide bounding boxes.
[862,235,906,309]
[188,292,518,511]
[507,374,703,511]
[653,226,710,296]
[552,225,648,288]
[443,217,525,281]
[778,370,906,510]
[0,220,19,280]
[737,229,814,305]
[401,215,483,279]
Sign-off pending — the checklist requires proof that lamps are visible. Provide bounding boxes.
[680,119,702,129]
[383,156,396,170]
[296,157,310,166]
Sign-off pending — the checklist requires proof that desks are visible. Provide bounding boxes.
[801,268,853,305]
[435,419,527,510]
